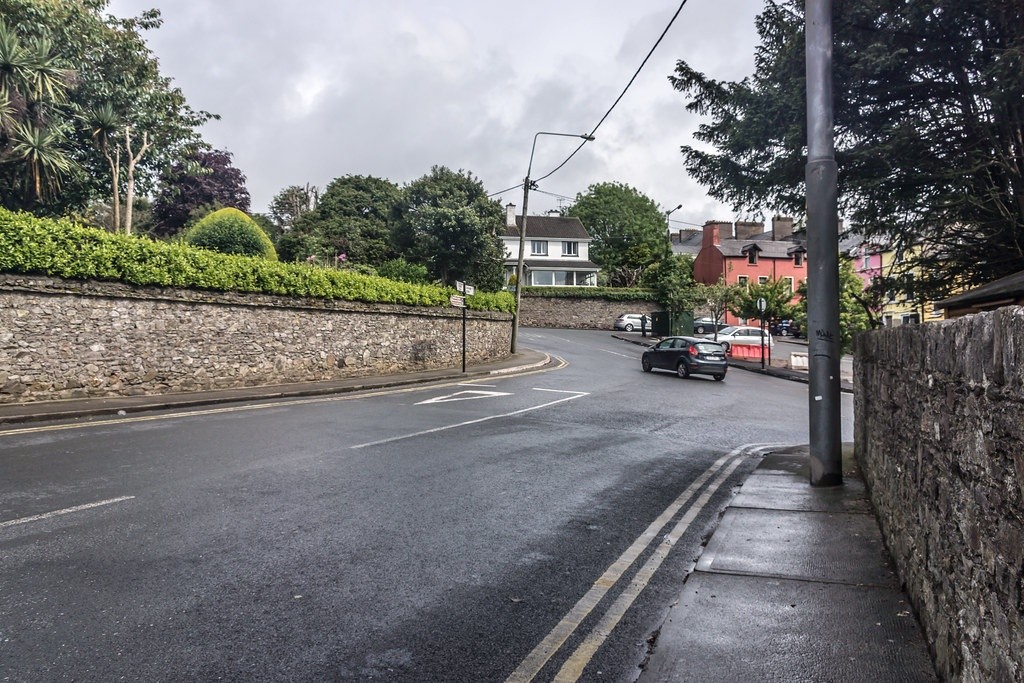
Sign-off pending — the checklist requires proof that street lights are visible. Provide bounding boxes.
[509,129,596,352]
[664,203,682,260]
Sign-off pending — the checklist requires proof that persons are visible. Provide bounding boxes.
[639,311,647,338]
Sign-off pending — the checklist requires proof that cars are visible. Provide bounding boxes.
[641,335,728,382]
[769,318,800,336]
[694,316,730,333]
[704,326,774,353]
[613,313,652,331]
